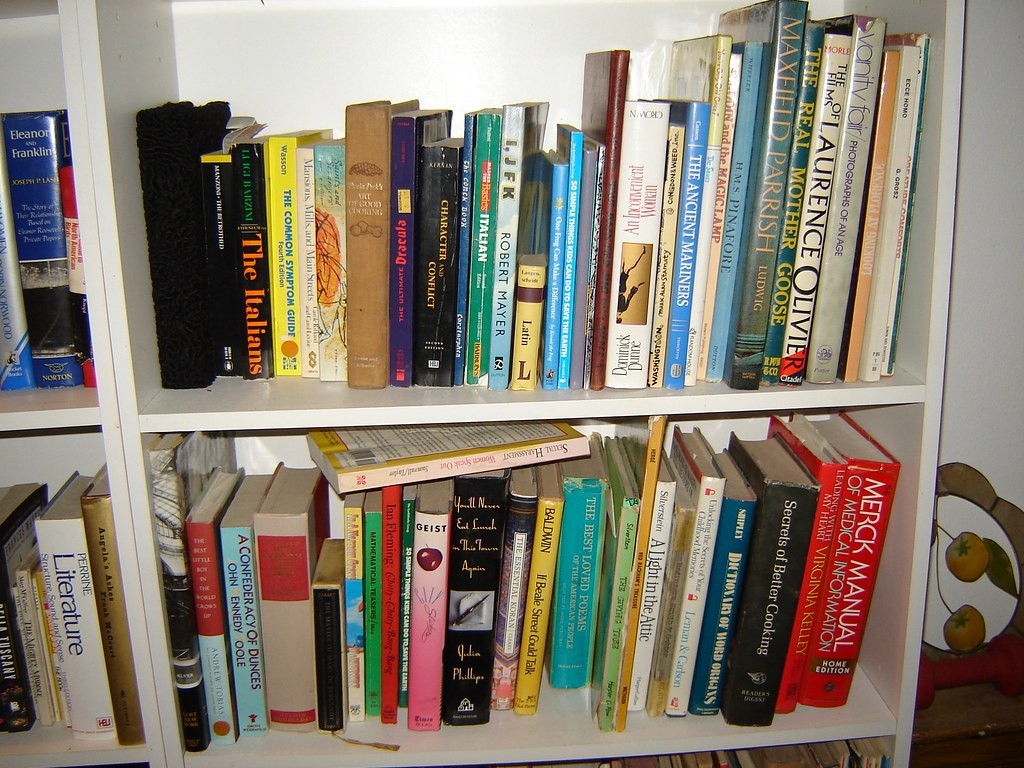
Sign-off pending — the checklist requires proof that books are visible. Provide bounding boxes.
[133,0,933,389]
[0,410,904,768]
[1,109,96,391]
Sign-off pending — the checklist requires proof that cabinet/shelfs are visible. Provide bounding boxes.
[76,0,966,768]
[0,0,167,768]
